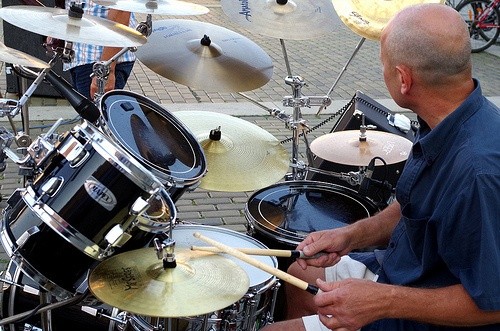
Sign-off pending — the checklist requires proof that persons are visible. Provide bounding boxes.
[259,3,500,331]
[46,0,137,125]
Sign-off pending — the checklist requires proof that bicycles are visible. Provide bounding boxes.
[445,0,500,53]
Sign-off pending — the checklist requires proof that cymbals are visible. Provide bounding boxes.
[0,5,149,47]
[331,0,447,43]
[309,129,414,167]
[173,111,290,193]
[92,0,210,15]
[133,19,274,94]
[0,42,51,70]
[87,245,250,318]
[220,0,344,40]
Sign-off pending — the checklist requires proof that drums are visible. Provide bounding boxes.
[108,224,280,331]
[82,89,207,205]
[109,187,178,259]
[0,118,165,300]
[0,257,112,331]
[244,179,382,276]
